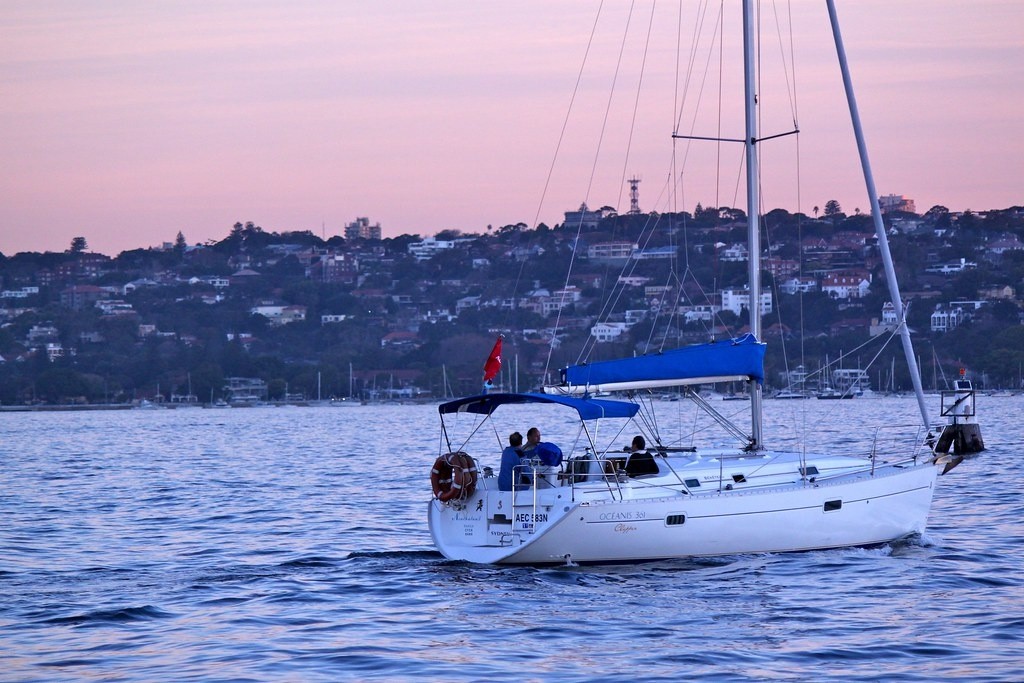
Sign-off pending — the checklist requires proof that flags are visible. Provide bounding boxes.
[960,368,965,374]
[483,336,502,382]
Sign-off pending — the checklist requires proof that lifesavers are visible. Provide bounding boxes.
[430,453,462,502]
[450,451,471,500]
[457,451,477,501]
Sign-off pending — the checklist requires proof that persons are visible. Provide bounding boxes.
[498,431,530,491]
[625,436,659,479]
[520,427,544,451]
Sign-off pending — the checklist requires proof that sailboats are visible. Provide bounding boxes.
[425,1,988,570]
[151,340,1024,412]
